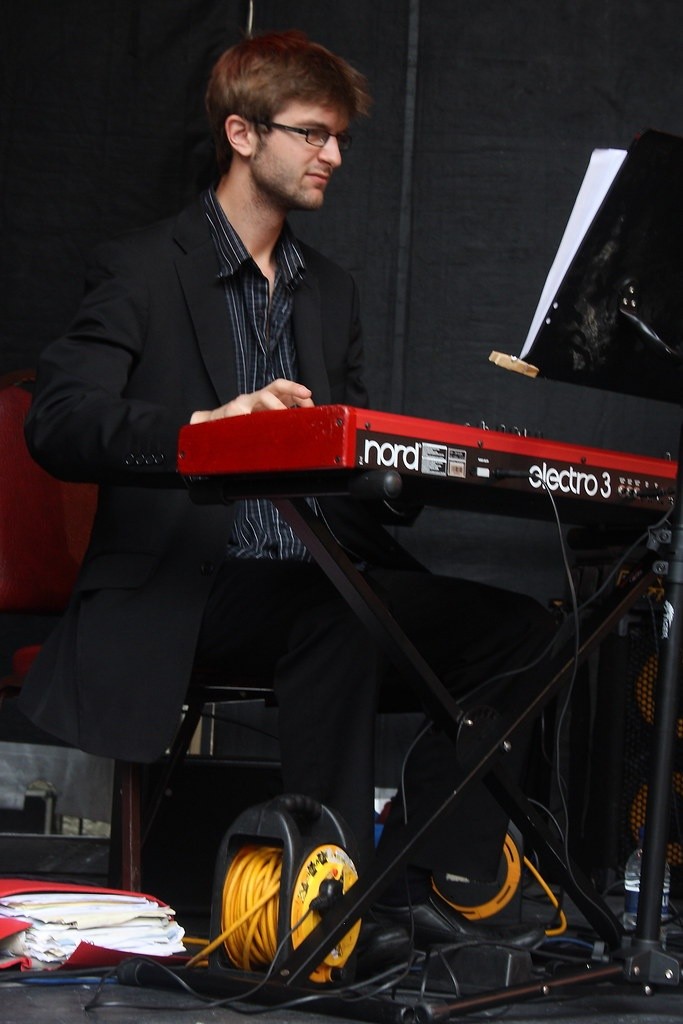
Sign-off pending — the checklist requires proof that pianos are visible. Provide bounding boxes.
[177,402,683,1007]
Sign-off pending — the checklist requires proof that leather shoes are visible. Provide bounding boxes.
[358,913,432,971]
[370,895,508,966]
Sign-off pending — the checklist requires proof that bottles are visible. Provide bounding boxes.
[624,826,669,956]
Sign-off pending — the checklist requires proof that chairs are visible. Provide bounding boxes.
[0,372,142,891]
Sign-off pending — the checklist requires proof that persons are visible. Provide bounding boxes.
[18,30,561,948]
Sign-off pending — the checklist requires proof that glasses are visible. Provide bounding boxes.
[246,117,353,153]
